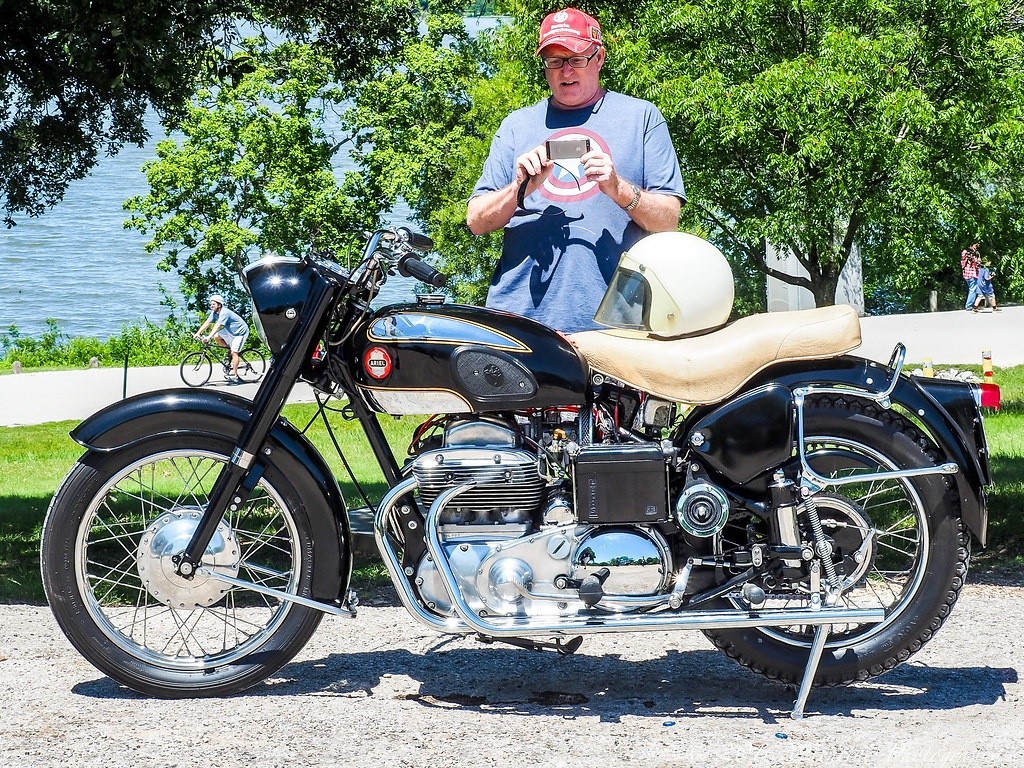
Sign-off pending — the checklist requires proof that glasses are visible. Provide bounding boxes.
[540,49,599,69]
[517,161,580,216]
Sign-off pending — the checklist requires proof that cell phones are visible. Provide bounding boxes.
[545,139,590,160]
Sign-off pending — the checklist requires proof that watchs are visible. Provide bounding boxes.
[621,180,642,212]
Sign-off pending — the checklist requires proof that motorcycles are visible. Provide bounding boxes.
[39,228,1001,721]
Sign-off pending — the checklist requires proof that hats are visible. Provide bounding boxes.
[536,7,602,57]
[210,295,223,305]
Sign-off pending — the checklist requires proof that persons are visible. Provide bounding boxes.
[193,294,249,383]
[465,7,689,335]
[960,241,1003,314]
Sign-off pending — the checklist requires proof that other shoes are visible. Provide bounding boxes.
[973,310,981,314]
[993,308,1002,311]
[966,307,975,310]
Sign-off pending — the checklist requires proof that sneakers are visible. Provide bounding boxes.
[224,373,238,382]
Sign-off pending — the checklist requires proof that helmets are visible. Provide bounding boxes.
[619,232,735,341]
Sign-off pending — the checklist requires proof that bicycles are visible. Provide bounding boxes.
[180,336,267,388]
